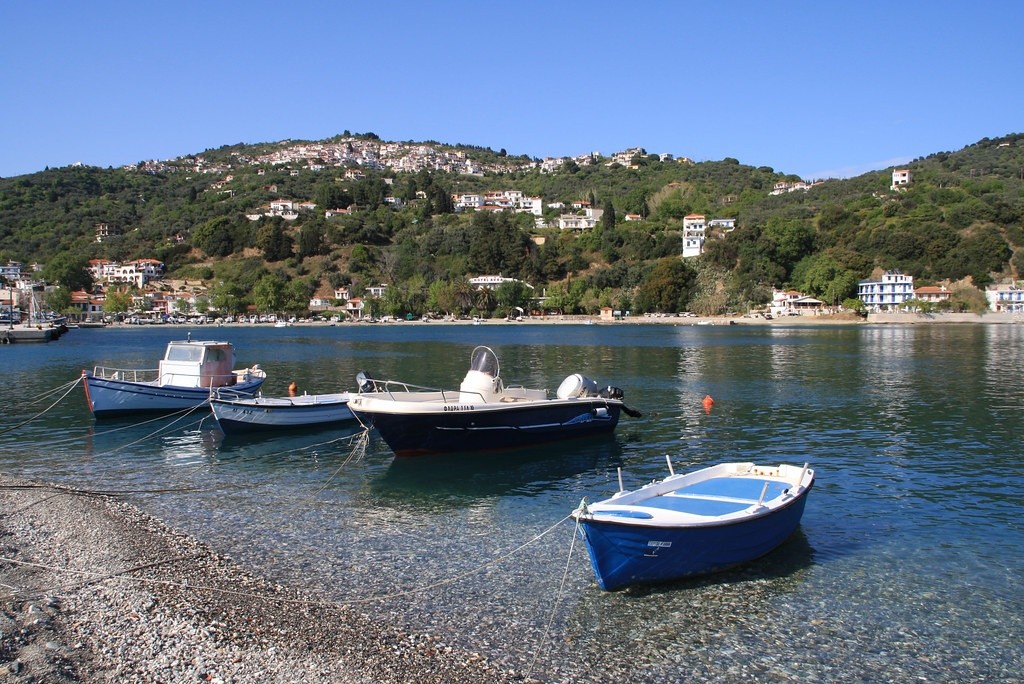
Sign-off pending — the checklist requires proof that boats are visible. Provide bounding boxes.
[345,346,624,463]
[82,333,267,420]
[209,383,358,434]
[570,454,816,590]
